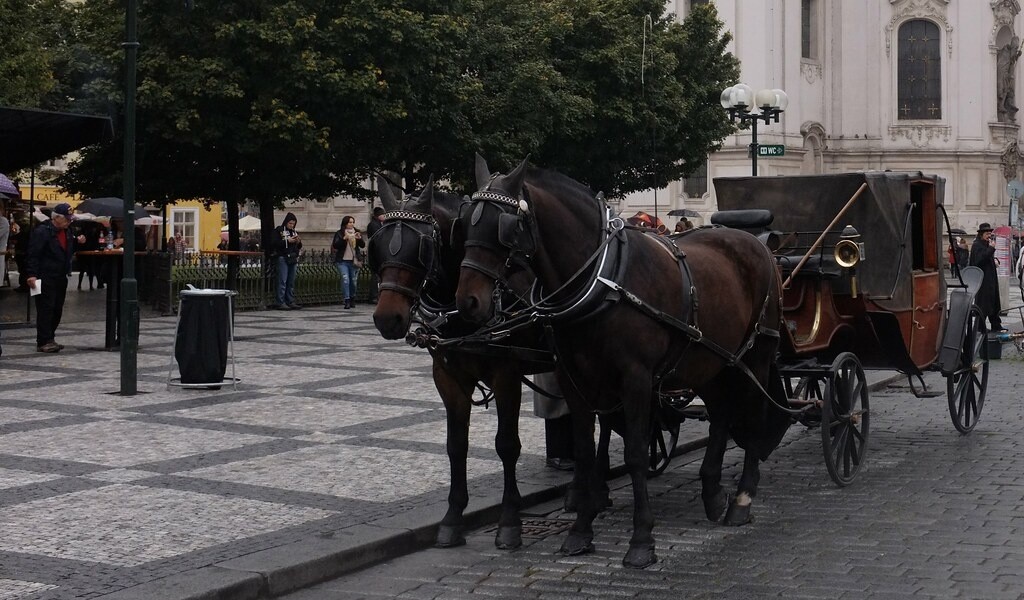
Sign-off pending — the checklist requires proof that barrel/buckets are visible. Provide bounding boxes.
[978,338,1001,359]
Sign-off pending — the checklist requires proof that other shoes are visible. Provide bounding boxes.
[48,340,65,349]
[977,329,990,333]
[288,303,302,310]
[997,329,1008,333]
[278,303,291,310]
[37,342,60,353]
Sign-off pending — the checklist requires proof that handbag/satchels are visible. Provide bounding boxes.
[352,255,364,269]
[283,243,297,265]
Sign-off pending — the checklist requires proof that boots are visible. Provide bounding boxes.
[350,296,355,308]
[345,299,351,309]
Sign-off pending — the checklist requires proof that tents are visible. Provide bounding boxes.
[1,108,114,325]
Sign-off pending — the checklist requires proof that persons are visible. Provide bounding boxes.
[948,237,1024,350]
[0,199,386,356]
[674,218,694,233]
[969,223,1009,333]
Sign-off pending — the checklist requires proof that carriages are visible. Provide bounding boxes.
[366,150,992,568]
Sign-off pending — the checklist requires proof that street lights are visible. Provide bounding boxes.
[720,84,787,176]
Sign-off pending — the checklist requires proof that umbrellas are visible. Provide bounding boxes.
[667,209,702,218]
[0,173,19,198]
[222,214,262,241]
[74,197,151,220]
[947,229,967,234]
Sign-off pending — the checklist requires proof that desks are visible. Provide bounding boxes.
[79,250,148,352]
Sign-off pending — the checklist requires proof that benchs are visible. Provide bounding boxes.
[775,231,854,276]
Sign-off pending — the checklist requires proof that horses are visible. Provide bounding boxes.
[454,150,784,570]
[367,170,613,550]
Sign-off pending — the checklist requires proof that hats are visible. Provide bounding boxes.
[177,232,181,238]
[374,207,386,216]
[54,204,80,220]
[977,223,994,232]
